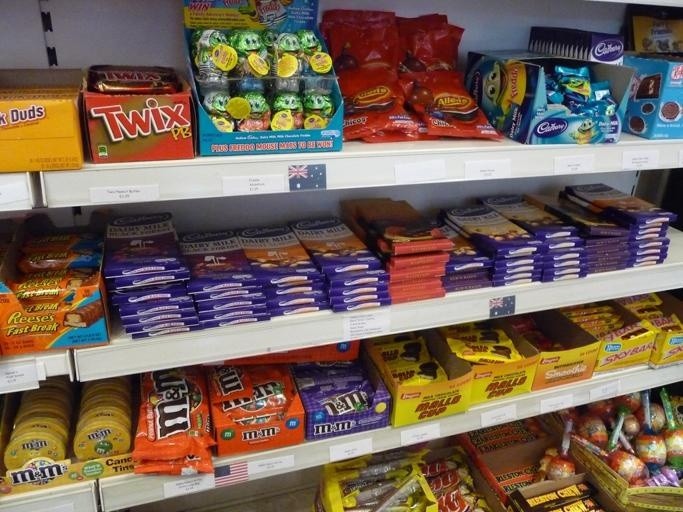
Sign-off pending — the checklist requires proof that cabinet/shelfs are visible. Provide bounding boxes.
[0,0,683,512]
